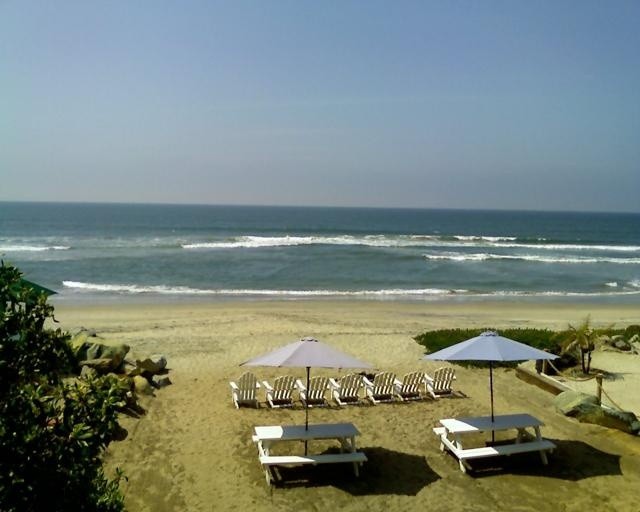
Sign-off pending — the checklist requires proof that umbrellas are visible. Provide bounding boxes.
[418,326,563,447]
[237,335,374,458]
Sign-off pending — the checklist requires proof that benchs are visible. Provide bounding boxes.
[453,440,557,473]
[258,452,368,485]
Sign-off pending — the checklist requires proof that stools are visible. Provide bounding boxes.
[432,427,449,452]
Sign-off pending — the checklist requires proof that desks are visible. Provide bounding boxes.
[252,422,362,488]
[439,413,549,476]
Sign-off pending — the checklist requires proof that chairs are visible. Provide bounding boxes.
[229,365,456,410]
